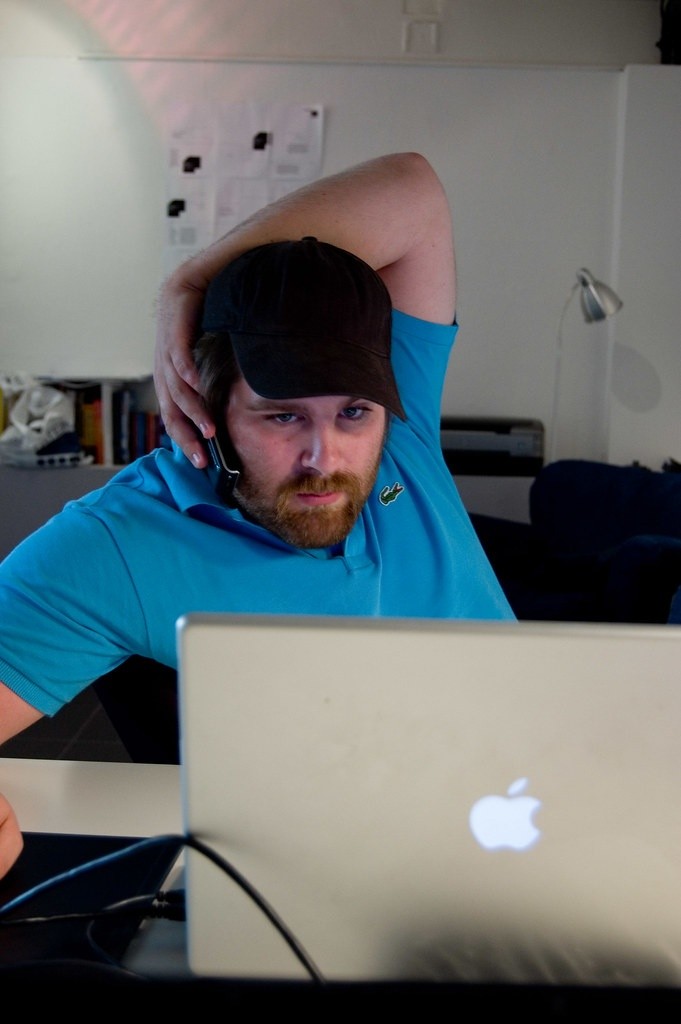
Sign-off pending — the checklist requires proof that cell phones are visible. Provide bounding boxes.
[199,428,240,503]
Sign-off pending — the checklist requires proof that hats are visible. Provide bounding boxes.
[199,235,410,424]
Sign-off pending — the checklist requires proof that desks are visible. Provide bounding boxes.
[0,755,182,1024]
[0,463,128,566]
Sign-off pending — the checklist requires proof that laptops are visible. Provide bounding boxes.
[171,612,681,992]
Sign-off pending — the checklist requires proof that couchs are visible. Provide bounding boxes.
[505,459,681,624]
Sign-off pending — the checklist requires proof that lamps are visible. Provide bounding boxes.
[550,268,624,463]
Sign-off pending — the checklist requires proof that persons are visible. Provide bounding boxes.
[1,151,517,882]
